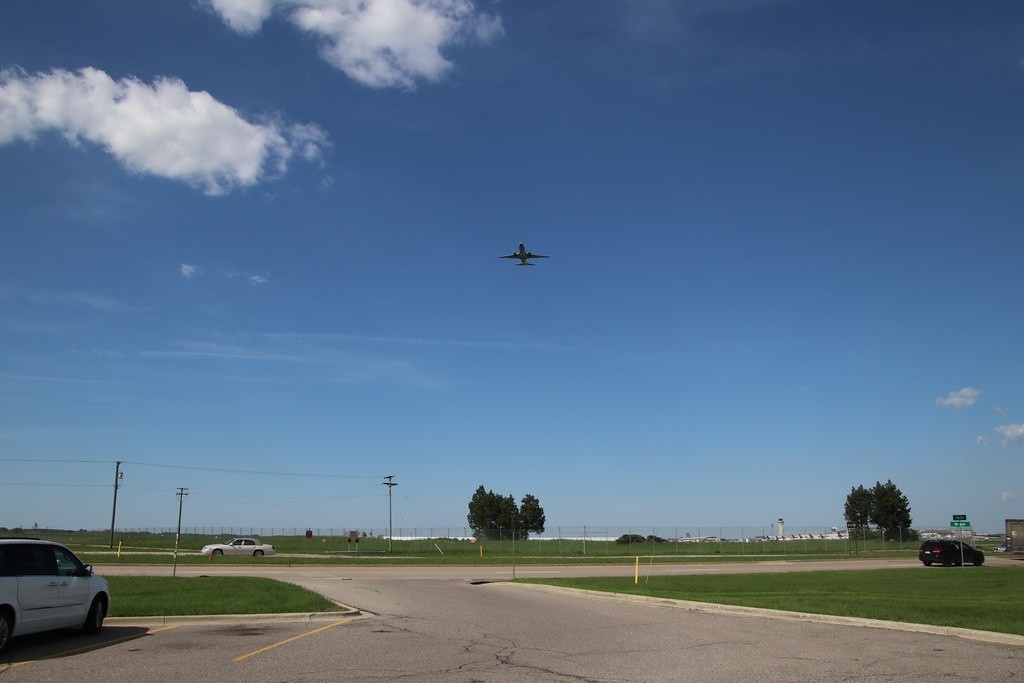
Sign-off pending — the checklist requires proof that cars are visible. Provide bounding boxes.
[201,537,277,559]
[992,542,1007,554]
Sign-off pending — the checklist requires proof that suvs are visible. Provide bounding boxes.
[0,536,111,651]
[919,539,986,568]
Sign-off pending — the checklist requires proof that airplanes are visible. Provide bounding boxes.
[498,243,550,268]
